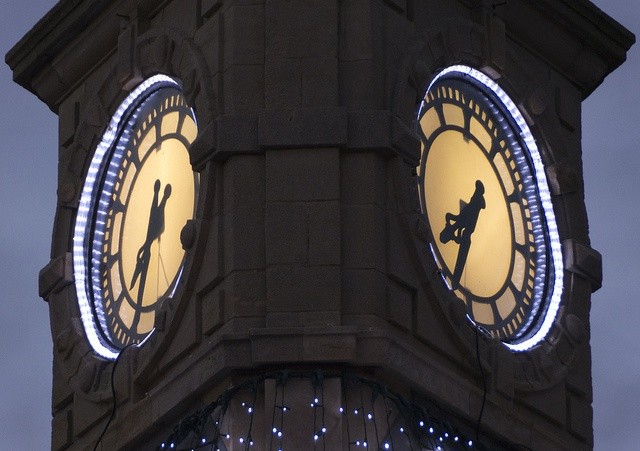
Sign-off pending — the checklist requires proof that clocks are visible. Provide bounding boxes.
[69,73,204,360]
[405,59,565,352]
